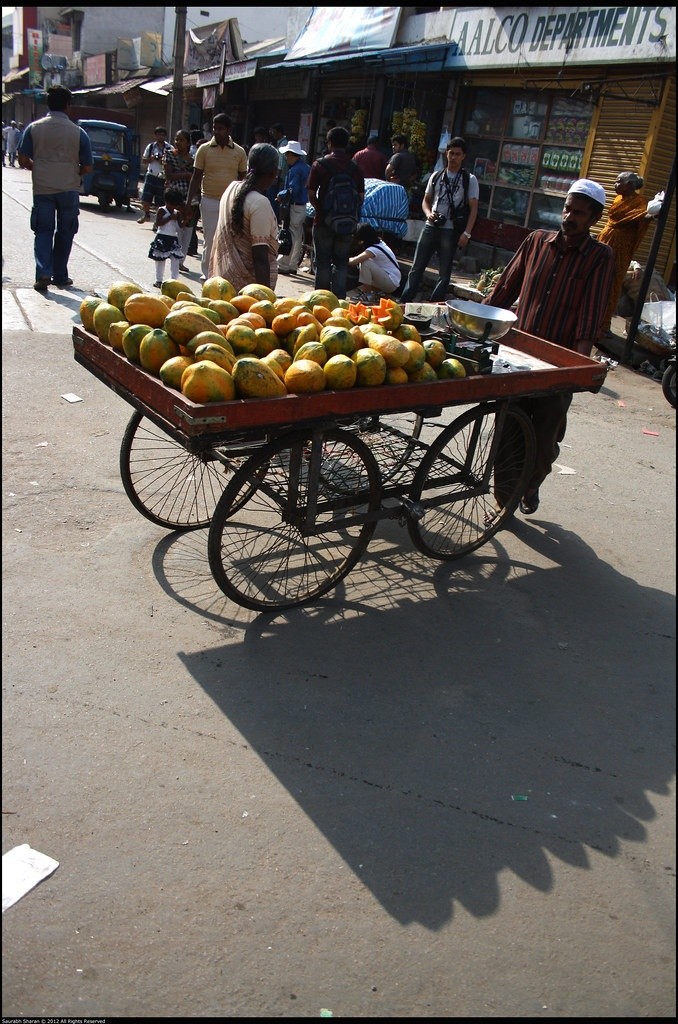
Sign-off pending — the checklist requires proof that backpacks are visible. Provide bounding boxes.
[315,157,360,236]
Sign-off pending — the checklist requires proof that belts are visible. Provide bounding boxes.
[290,201,306,205]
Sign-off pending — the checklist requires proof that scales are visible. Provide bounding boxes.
[440,300,518,375]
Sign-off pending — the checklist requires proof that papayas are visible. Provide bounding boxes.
[77,276,467,403]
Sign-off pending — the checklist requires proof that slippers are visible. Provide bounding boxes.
[519,488,540,514]
[482,503,506,526]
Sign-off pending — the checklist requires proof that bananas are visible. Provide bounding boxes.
[351,109,368,135]
[391,108,426,149]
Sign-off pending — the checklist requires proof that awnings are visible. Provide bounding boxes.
[195,48,288,92]
[257,39,453,87]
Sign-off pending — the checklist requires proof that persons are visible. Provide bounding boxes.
[478,177,619,527]
[394,137,479,303]
[123,113,422,301]
[18,86,95,289]
[2,120,24,167]
[590,172,655,317]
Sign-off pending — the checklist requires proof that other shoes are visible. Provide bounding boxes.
[12,164,15,167]
[153,222,158,231]
[350,292,371,304]
[179,264,189,272]
[153,282,162,288]
[367,291,386,304]
[200,273,208,281]
[52,276,73,285]
[3,163,6,166]
[34,277,51,290]
[137,214,150,223]
[289,269,297,275]
[278,268,290,275]
[187,246,198,256]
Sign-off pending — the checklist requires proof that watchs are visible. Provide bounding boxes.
[464,231,471,239]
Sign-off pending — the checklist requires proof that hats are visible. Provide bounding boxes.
[568,178,606,208]
[278,141,307,155]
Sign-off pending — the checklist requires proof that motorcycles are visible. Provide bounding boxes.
[76,120,140,212]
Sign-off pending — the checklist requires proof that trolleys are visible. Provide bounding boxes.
[72,298,610,614]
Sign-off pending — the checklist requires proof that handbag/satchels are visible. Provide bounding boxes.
[184,194,200,205]
[277,229,292,255]
[452,206,477,235]
